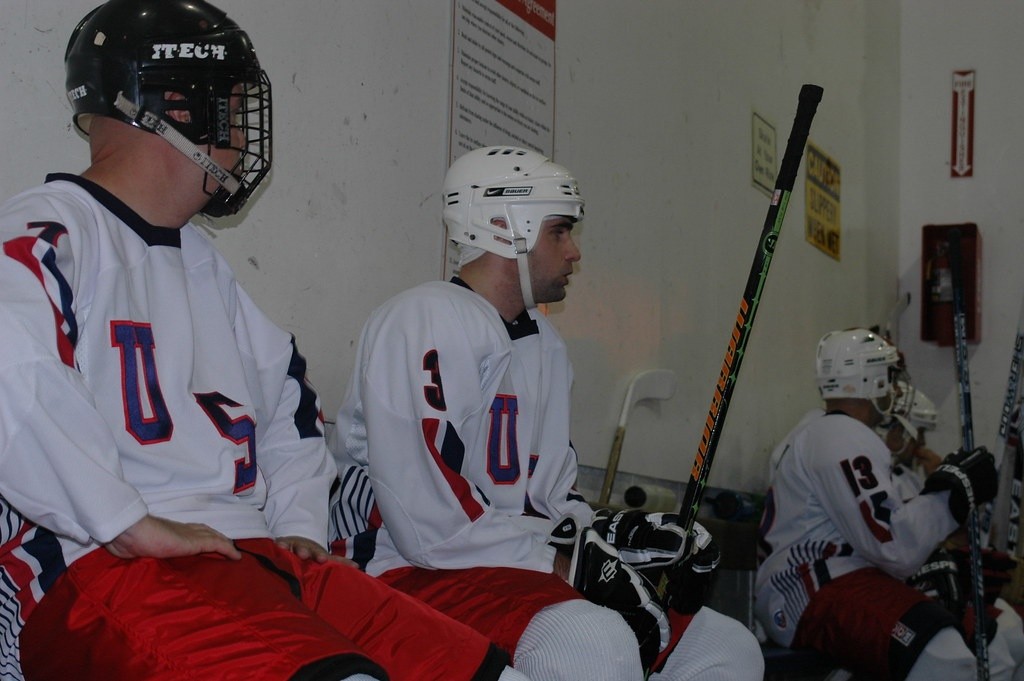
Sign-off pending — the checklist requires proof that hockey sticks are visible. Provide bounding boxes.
[875,276,912,365]
[598,370,676,505]
[949,225,1024,681]
[645,82,822,681]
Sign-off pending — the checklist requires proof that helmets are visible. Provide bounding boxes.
[881,380,938,442]
[443,145,584,257]
[815,329,899,413]
[66,0,273,217]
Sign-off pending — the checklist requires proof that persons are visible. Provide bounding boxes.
[0,0,527,681]
[754,328,1024,681]
[328,145,765,681]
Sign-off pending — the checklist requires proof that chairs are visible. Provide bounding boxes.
[695,518,821,680]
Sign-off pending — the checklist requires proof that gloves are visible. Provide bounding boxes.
[925,554,973,614]
[918,444,1000,524]
[564,502,720,667]
